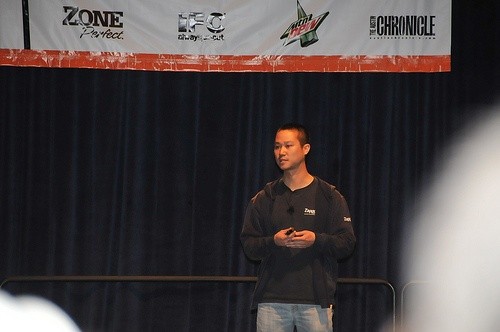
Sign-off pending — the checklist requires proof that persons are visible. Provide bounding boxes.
[239,120,357,331]
